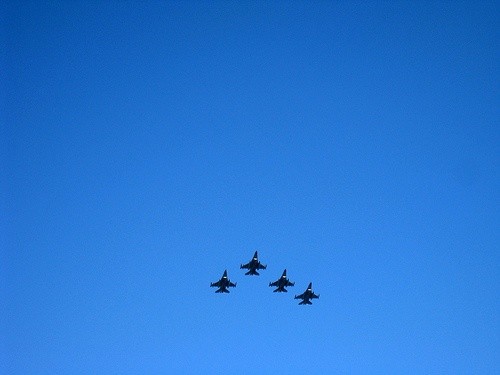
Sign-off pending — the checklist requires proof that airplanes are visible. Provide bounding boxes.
[294,282,320,305]
[268,269,296,293]
[210,270,237,293]
[240,250,267,276]
